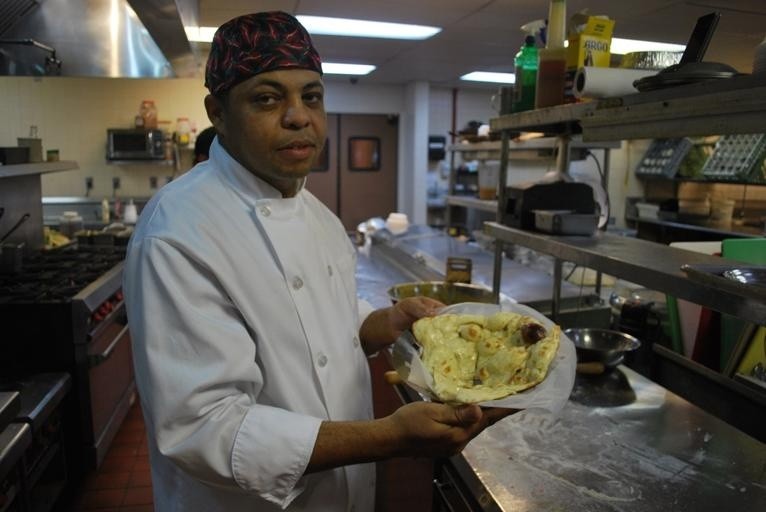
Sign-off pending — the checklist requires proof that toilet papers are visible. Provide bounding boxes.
[572,65,658,100]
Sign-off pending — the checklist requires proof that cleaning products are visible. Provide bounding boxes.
[513,17,548,111]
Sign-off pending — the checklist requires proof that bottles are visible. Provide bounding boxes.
[511,35,540,114]
[533,0,569,109]
[101,195,138,226]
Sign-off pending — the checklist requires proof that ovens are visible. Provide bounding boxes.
[1,283,139,472]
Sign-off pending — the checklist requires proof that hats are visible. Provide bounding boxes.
[203,11,324,98]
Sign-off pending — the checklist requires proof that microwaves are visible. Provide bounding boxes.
[106,127,165,160]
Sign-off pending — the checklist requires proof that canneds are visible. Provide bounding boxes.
[47,149,60,163]
[135,115,145,130]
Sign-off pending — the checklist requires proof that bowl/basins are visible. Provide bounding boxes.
[385,279,499,330]
[560,327,641,380]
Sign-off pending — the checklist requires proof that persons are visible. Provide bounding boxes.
[118,10,518,510]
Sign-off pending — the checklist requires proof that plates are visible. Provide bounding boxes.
[391,326,440,403]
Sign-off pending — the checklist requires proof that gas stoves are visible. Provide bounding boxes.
[0,242,128,303]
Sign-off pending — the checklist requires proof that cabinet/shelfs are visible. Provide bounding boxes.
[482,69,766,326]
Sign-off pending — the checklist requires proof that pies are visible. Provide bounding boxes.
[410,310,561,405]
